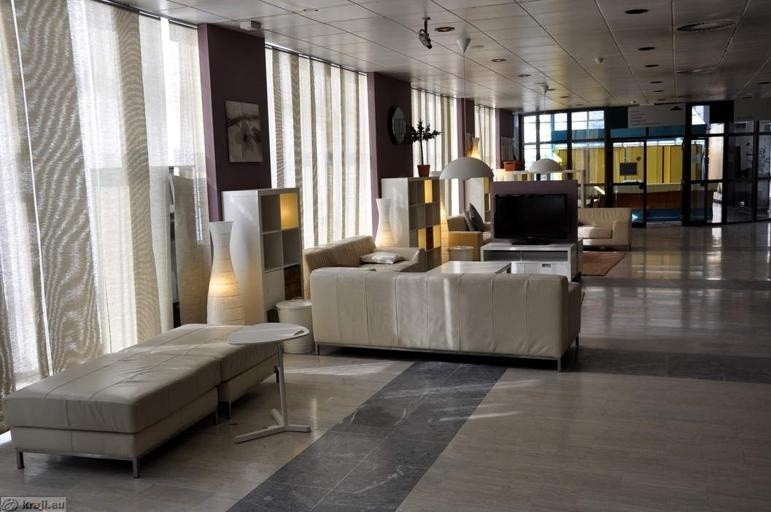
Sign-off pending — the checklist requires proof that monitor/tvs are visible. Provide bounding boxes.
[493,193,569,245]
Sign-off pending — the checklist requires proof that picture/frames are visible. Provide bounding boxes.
[225,99,264,164]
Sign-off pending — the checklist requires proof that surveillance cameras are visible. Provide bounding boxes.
[418,33,433,49]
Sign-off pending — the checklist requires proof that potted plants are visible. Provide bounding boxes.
[401,118,444,177]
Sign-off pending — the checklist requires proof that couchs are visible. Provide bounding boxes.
[309,267,581,372]
[578,207,635,250]
[303,235,426,302]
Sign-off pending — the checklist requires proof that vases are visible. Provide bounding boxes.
[503,161,521,171]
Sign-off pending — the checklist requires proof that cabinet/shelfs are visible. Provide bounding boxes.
[221,187,304,322]
[578,238,583,275]
[480,242,577,283]
[464,169,491,223]
[380,176,440,266]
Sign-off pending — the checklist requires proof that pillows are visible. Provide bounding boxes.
[360,252,409,264]
[469,203,483,228]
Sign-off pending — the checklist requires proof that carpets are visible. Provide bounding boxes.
[631,209,713,220]
[581,250,627,277]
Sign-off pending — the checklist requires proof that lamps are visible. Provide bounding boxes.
[470,138,481,160]
[374,199,396,248]
[205,221,245,326]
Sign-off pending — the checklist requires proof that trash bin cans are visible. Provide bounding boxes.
[446,245,475,261]
[275,299,314,354]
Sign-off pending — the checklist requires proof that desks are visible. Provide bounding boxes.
[226,322,311,444]
[614,183,713,210]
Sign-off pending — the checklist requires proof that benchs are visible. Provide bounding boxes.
[6,323,278,478]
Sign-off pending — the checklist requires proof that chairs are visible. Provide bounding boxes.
[441,214,490,261]
